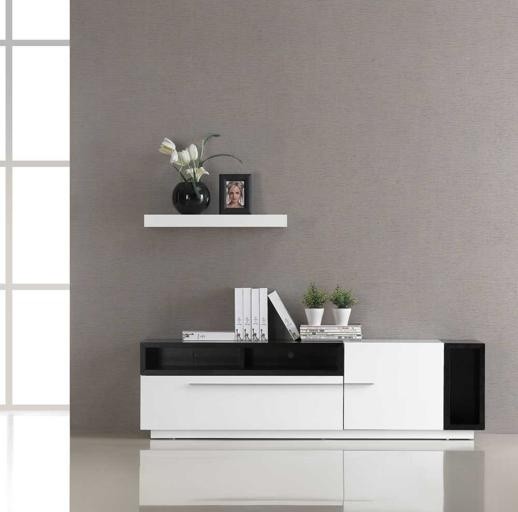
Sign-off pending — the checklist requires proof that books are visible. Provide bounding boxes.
[181,288,362,343]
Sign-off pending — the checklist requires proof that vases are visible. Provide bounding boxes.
[171,181,210,215]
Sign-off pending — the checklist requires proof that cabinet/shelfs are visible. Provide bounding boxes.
[137,444,487,510]
[135,336,486,441]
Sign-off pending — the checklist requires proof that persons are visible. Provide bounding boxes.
[226,181,244,208]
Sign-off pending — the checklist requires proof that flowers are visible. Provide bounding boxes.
[159,129,244,181]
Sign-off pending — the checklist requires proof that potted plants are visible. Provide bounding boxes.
[300,282,358,328]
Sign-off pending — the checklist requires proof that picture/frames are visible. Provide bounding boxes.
[218,173,250,214]
